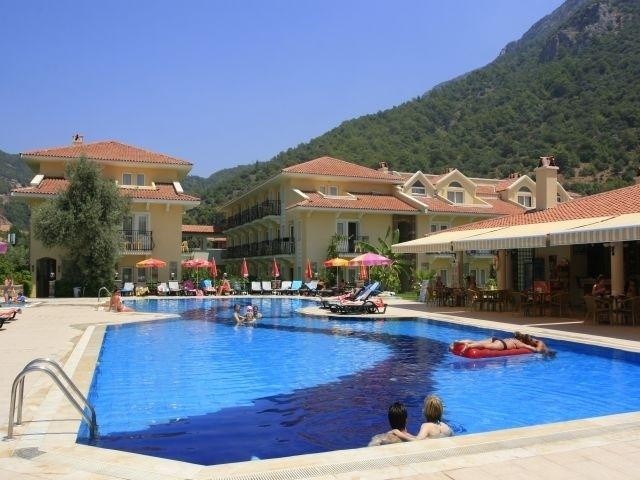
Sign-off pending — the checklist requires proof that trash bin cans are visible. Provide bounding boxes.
[72,286,83,297]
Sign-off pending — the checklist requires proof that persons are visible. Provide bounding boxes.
[449,331,537,356]
[215,276,230,296]
[3,273,25,305]
[592,276,608,308]
[367,402,414,446]
[319,289,356,302]
[392,395,452,441]
[108,289,135,312]
[436,276,476,306]
[524,334,548,353]
[232,303,263,326]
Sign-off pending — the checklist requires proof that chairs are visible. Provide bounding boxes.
[120,279,322,295]
[316,282,388,315]
[431,283,640,321]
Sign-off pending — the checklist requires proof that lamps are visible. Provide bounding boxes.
[603,241,616,255]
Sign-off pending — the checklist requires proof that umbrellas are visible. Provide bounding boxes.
[348,252,391,285]
[360,260,368,286]
[241,257,249,290]
[304,259,312,283]
[210,257,217,287]
[183,259,212,288]
[135,258,166,286]
[271,257,280,289]
[322,257,349,287]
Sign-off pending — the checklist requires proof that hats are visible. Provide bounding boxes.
[247,306,253,312]
